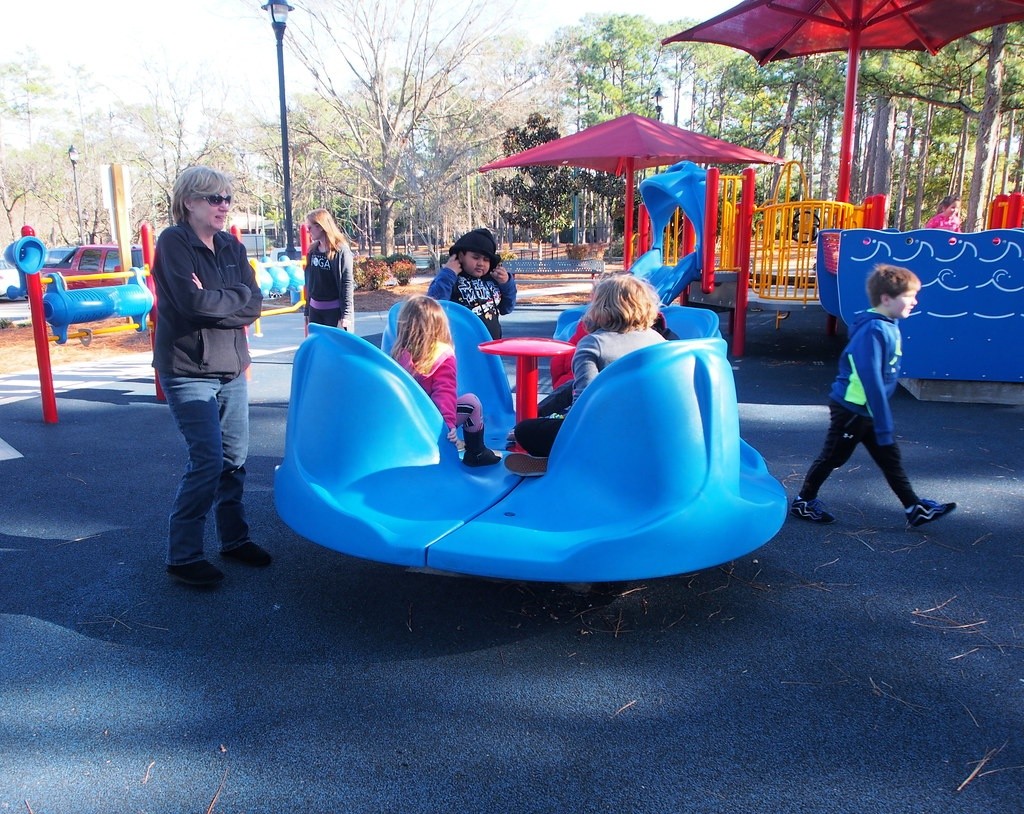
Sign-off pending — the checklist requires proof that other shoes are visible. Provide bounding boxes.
[219,542,273,570]
[167,560,224,585]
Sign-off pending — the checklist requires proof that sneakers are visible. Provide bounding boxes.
[904,498,956,527]
[504,453,548,476]
[790,499,836,525]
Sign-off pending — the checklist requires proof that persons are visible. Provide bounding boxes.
[927,195,964,233]
[390,293,502,466]
[787,265,958,527]
[150,165,270,588]
[304,208,354,342]
[426,227,517,343]
[503,268,689,479]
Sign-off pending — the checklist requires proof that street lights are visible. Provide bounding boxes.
[67,142,88,244]
[261,0,299,254]
[653,88,667,122]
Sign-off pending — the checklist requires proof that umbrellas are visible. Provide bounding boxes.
[660,0,1024,207]
[477,110,782,271]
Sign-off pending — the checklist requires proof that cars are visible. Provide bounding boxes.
[41,245,156,290]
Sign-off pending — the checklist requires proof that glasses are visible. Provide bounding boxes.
[202,194,231,207]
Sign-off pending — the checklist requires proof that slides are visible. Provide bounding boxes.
[553,247,698,342]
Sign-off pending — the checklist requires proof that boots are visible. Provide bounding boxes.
[463,424,501,466]
